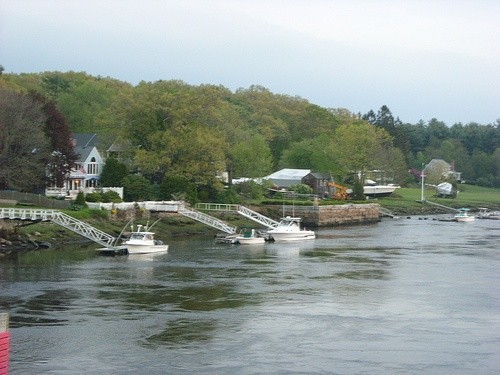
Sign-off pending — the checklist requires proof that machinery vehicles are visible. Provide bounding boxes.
[324,180,349,201]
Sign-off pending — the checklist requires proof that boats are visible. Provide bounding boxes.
[265,195,318,243]
[454,207,472,223]
[91,213,168,256]
[214,225,266,246]
[485,210,500,219]
[475,207,489,218]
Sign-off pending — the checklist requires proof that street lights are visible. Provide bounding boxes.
[419,162,427,200]
